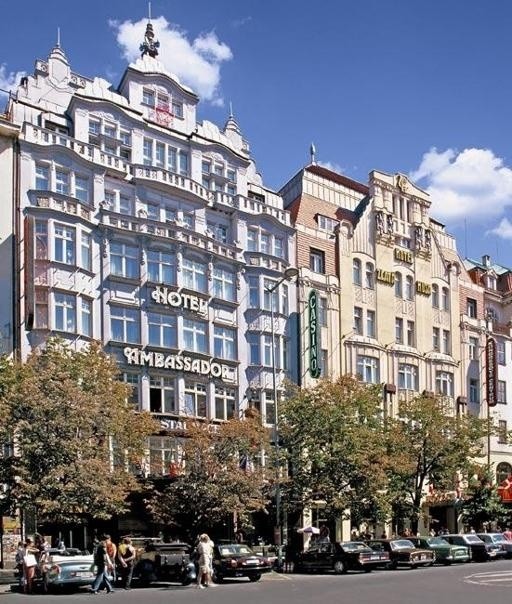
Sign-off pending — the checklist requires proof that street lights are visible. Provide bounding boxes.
[265,264,302,569]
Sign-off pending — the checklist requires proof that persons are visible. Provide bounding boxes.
[13,532,217,595]
[351,526,512,542]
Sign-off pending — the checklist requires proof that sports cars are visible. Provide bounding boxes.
[120,534,197,586]
[20,546,113,589]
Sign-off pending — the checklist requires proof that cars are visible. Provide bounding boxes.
[475,532,512,554]
[299,540,390,574]
[439,532,501,560]
[405,535,469,566]
[365,537,436,571]
[193,543,273,583]
